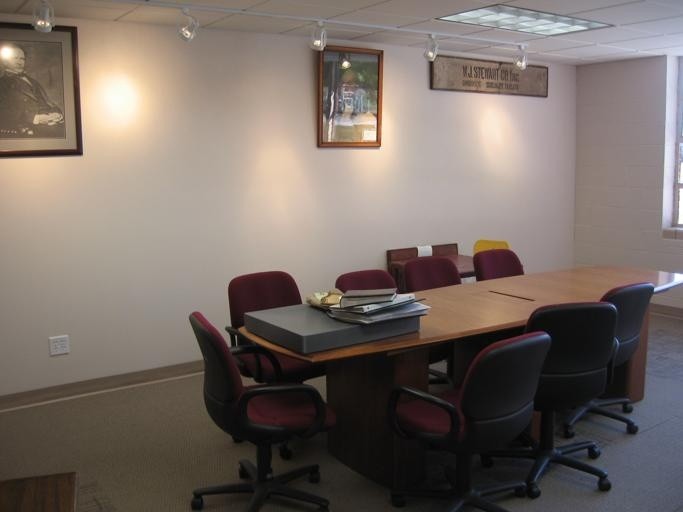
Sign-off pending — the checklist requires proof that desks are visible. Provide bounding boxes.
[0,470,81,511]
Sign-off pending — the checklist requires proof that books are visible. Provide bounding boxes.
[325,287,431,325]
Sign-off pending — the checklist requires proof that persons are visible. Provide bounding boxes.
[0,42,65,138]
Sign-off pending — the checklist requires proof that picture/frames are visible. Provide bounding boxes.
[314,44,385,150]
[0,23,82,160]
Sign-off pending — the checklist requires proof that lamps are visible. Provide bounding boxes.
[1,0,531,72]
